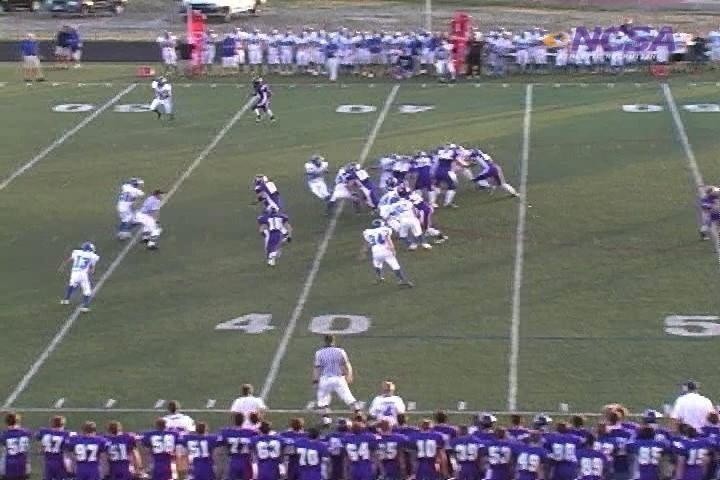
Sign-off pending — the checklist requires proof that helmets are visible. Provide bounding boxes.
[346,162,360,172]
[386,177,399,192]
[128,178,145,189]
[312,155,324,164]
[158,76,168,86]
[371,218,385,227]
[535,414,553,426]
[82,241,96,253]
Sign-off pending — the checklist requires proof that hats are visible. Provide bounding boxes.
[479,414,496,425]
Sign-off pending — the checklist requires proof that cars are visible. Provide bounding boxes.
[181,0,266,25]
[28,0,128,18]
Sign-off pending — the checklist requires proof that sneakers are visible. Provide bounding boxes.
[408,232,449,252]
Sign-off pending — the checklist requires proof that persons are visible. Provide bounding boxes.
[22,32,46,83]
[706,187,719,253]
[117,177,145,240]
[155,25,718,84]
[53,24,83,68]
[135,188,167,250]
[252,77,277,125]
[312,335,363,428]
[694,183,711,241]
[254,143,520,290]
[149,76,173,120]
[61,240,100,313]
[1,382,720,479]
